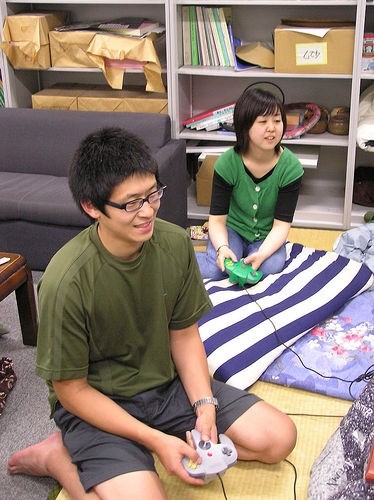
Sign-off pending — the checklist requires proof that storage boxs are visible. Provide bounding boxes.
[274,25,354,75]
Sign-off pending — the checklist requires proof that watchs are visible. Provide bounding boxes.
[191,397,220,413]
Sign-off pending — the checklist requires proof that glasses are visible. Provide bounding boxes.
[104,179,168,213]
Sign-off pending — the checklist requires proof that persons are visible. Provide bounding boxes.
[6,124,298,500]
[195,88,305,280]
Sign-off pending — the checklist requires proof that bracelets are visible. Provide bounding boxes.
[216,244,231,256]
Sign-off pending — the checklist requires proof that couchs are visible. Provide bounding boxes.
[0,107,186,276]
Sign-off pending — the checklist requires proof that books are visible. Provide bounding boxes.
[181,6,259,71]
[183,102,236,131]
[102,20,160,37]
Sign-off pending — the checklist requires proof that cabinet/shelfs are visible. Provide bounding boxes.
[172,0,374,228]
[0,1,170,138]
